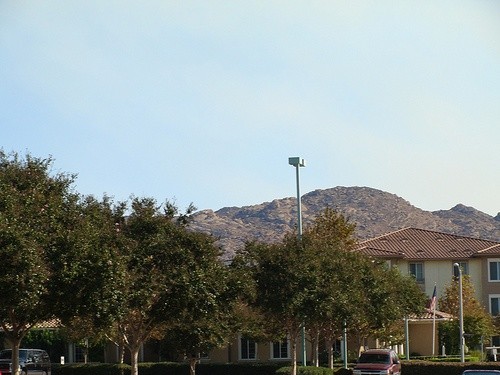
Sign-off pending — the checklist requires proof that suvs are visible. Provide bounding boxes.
[0,349,51,375]
[352,348,402,375]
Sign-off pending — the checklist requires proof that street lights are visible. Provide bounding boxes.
[453,262,466,363]
[289,156,309,367]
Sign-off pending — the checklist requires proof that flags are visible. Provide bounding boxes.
[430,288,436,312]
